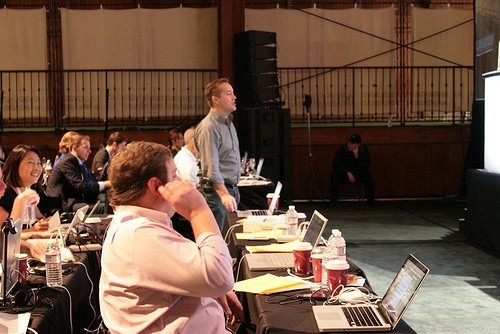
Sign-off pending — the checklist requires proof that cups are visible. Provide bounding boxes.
[311,254,325,283]
[266,192,280,211]
[292,242,314,275]
[18,254,28,282]
[325,259,349,294]
[85,216,102,238]
[298,213,306,229]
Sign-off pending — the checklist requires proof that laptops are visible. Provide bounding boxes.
[246,209,329,270]
[237,181,282,217]
[25,199,101,244]
[98,163,108,182]
[240,157,264,181]
[313,253,430,332]
[241,152,248,176]
[0,219,23,301]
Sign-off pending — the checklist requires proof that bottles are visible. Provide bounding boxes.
[46,159,51,169]
[41,157,47,169]
[322,239,338,285]
[45,239,63,286]
[330,231,347,260]
[325,228,339,255]
[285,205,298,235]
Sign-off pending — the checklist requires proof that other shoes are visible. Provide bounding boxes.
[367,200,379,207]
[326,202,338,207]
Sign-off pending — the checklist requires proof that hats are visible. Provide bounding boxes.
[348,134,361,143]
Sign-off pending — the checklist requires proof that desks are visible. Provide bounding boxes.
[0,166,417,334]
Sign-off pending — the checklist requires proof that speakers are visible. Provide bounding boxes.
[233,29,291,200]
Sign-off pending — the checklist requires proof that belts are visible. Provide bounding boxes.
[206,181,237,189]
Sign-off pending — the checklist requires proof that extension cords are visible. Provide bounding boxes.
[70,243,101,252]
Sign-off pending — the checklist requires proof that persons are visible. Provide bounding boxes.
[46,131,111,210]
[193,79,241,232]
[328,133,377,209]
[0,145,53,231]
[174,128,199,188]
[0,160,44,259]
[92,131,128,171]
[99,142,246,334]
[170,131,184,156]
